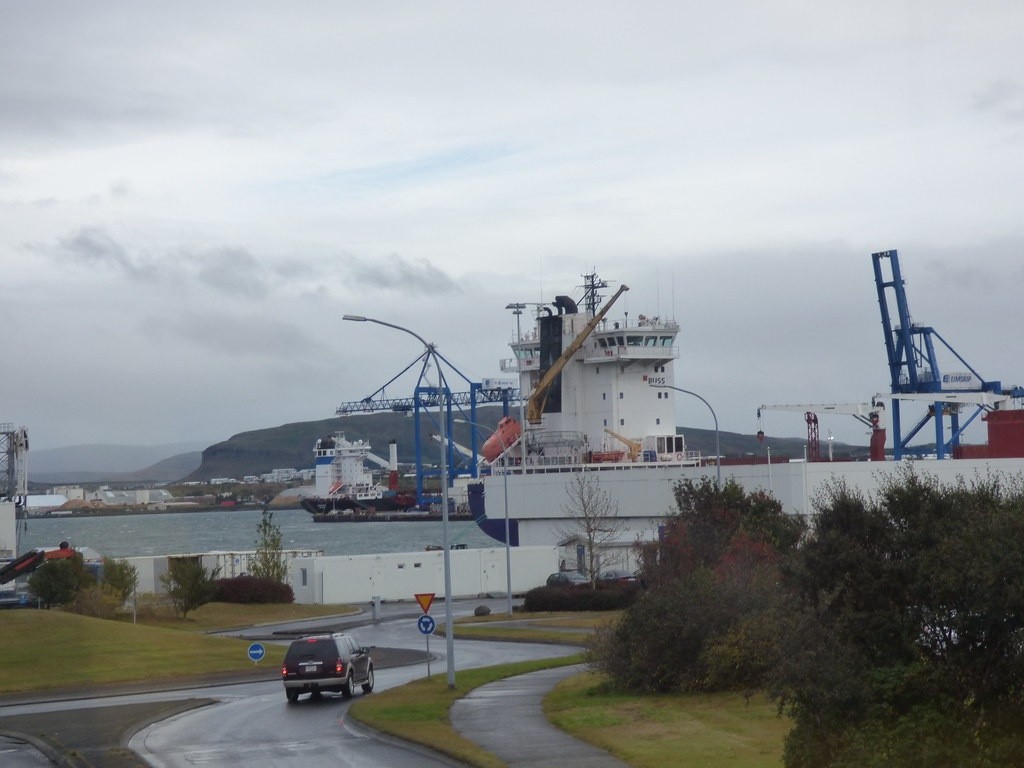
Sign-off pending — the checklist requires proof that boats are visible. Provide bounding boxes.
[298,490,416,514]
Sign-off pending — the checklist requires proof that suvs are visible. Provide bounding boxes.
[282,632,374,704]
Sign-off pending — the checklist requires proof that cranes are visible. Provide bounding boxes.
[333,343,521,510]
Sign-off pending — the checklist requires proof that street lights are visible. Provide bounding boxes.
[505,302,527,475]
[452,418,514,618]
[342,314,456,690]
[647,384,720,493]
